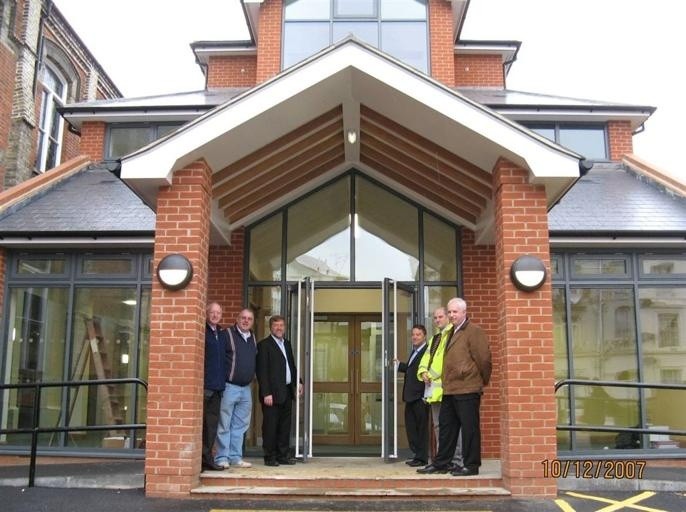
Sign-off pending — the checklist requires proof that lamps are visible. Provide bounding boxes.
[155,252,194,290]
[509,254,548,292]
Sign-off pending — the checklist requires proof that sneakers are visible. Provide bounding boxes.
[217,462,230,468]
[231,461,251,467]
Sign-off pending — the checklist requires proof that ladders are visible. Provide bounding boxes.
[48,311,126,448]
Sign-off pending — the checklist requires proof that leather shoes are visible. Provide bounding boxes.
[406,459,425,466]
[453,466,479,476]
[279,458,296,465]
[203,464,224,471]
[265,460,279,466]
[417,464,448,474]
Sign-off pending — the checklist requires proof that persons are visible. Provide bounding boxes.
[216,309,258,468]
[416,297,492,476]
[392,325,429,467]
[203,302,227,469]
[417,307,463,470]
[256,315,303,468]
